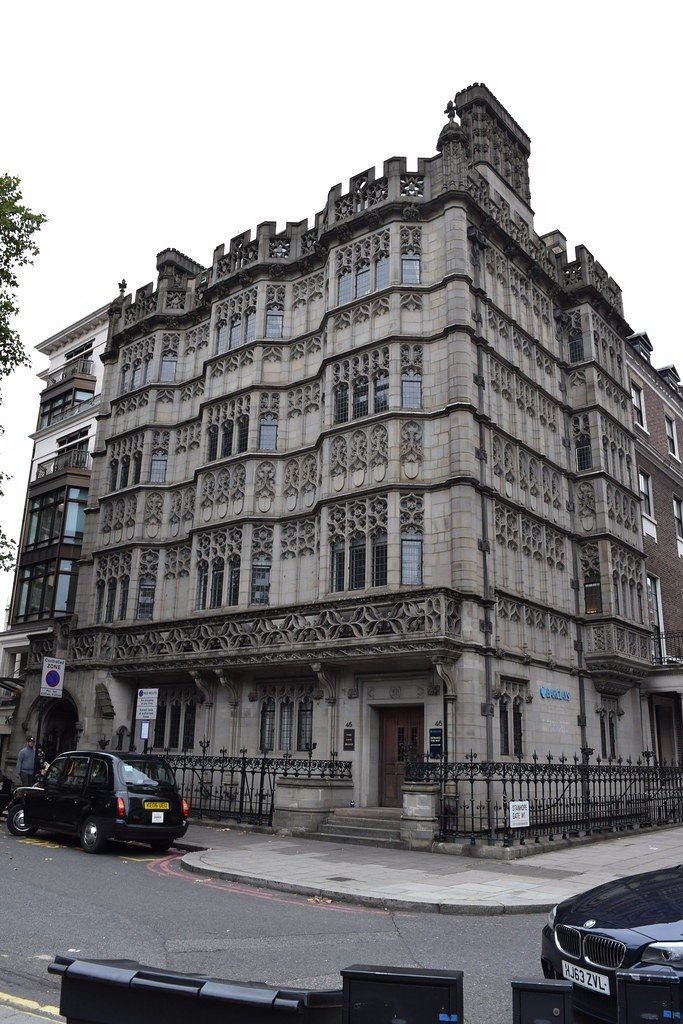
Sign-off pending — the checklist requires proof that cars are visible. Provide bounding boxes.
[0,771,11,814]
[540,864,683,1024]
[6,750,188,855]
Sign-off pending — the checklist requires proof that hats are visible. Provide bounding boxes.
[27,737,35,742]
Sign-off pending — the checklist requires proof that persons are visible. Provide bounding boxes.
[16,736,36,788]
[36,745,46,770]
